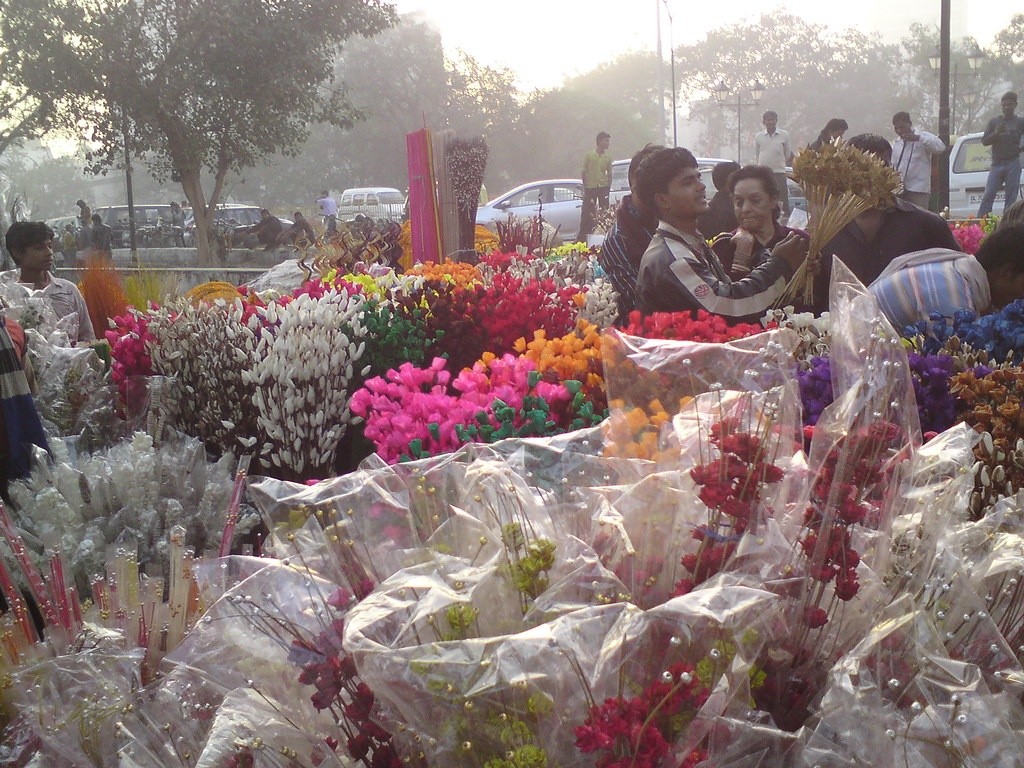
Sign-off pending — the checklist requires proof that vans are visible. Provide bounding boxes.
[338,188,406,222]
[92,205,173,247]
[610,157,734,191]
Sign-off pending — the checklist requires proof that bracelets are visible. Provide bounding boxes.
[993,130,999,138]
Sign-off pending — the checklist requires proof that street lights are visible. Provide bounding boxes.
[963,86,978,134]
[928,44,986,134]
[713,79,766,164]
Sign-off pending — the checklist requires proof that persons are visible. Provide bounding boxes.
[169,200,194,247]
[866,203,1024,339]
[5,221,95,348]
[246,209,283,251]
[754,111,791,220]
[314,190,338,231]
[0,296,52,642]
[819,134,961,311]
[276,212,315,250]
[61,200,113,268]
[810,119,849,154]
[600,145,823,328]
[891,111,945,210]
[976,91,1024,219]
[572,132,614,245]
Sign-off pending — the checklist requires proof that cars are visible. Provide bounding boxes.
[519,188,580,206]
[401,181,489,222]
[476,178,631,244]
[948,129,1024,218]
[699,168,807,215]
[157,203,296,247]
[47,216,84,252]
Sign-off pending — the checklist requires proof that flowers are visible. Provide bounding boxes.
[0,134,1024,768]
